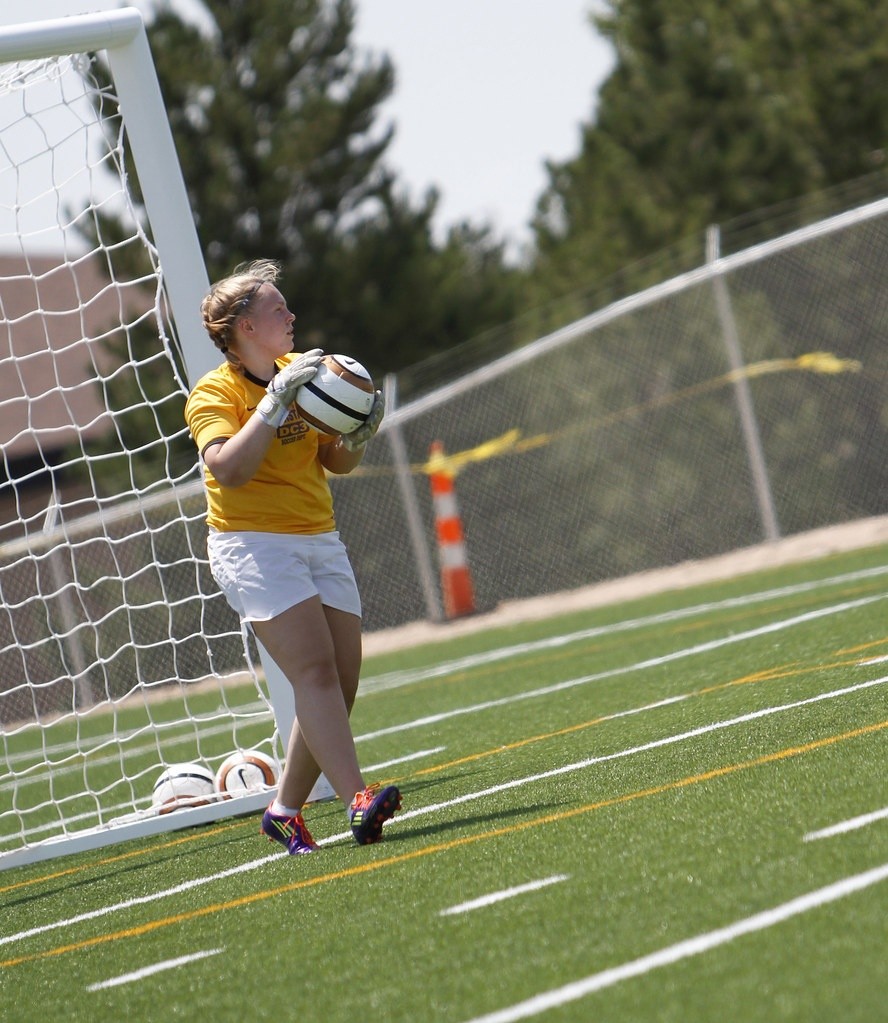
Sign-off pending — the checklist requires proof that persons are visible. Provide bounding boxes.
[184,265,404,856]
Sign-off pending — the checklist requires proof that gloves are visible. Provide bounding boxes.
[255,347,324,429]
[341,390,386,452]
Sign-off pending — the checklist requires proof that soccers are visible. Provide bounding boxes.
[151,762,216,816]
[214,751,282,818]
[295,354,377,436]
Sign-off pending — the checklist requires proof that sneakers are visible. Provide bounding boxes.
[259,801,321,855]
[348,783,403,845]
